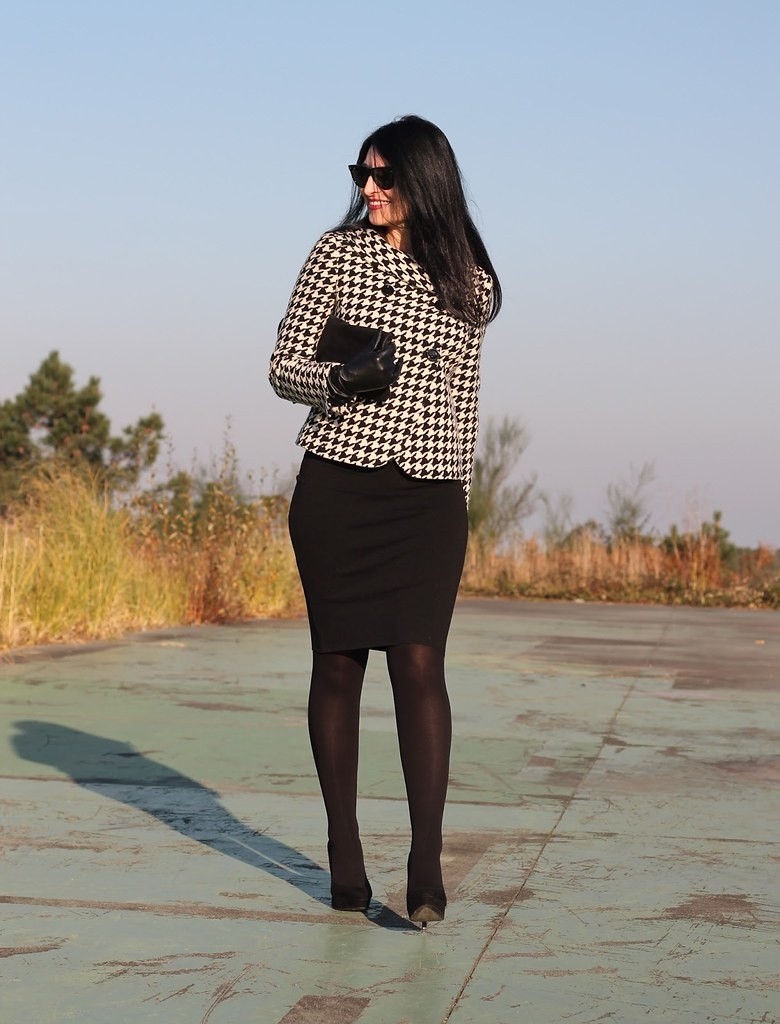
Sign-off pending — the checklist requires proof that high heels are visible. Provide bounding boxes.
[327,840,373,911]
[406,851,447,929]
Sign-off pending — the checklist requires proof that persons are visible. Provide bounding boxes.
[270,116,502,929]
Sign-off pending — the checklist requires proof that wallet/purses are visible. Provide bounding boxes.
[315,314,394,365]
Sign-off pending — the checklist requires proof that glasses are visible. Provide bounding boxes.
[348,162,398,191]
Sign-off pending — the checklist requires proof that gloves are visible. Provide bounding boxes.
[327,326,404,406]
